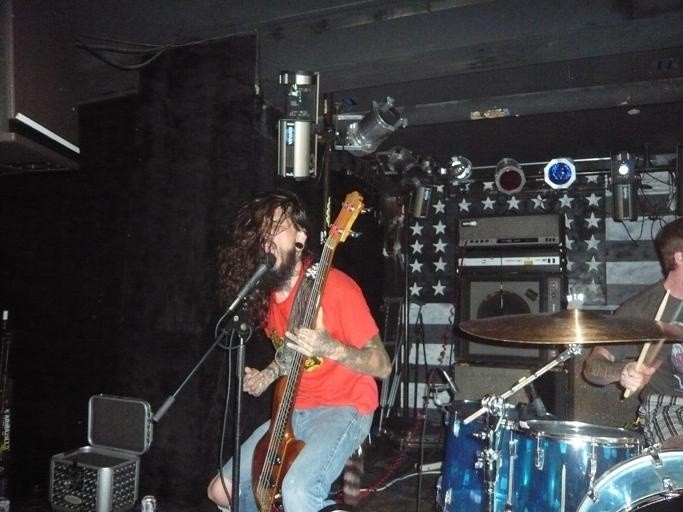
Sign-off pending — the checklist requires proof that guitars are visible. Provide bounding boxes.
[253,192,367,512]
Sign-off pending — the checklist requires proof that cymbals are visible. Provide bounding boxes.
[459,308,683,344]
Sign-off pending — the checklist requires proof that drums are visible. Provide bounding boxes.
[506,419,646,512]
[576,449,683,512]
[437,399,554,512]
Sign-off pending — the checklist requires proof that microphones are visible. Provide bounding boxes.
[228,254,276,312]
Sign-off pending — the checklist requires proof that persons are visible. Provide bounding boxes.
[581,218,683,451]
[206,189,394,512]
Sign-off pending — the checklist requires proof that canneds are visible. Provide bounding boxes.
[141,495,157,512]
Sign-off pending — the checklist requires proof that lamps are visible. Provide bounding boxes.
[492,155,577,195]
